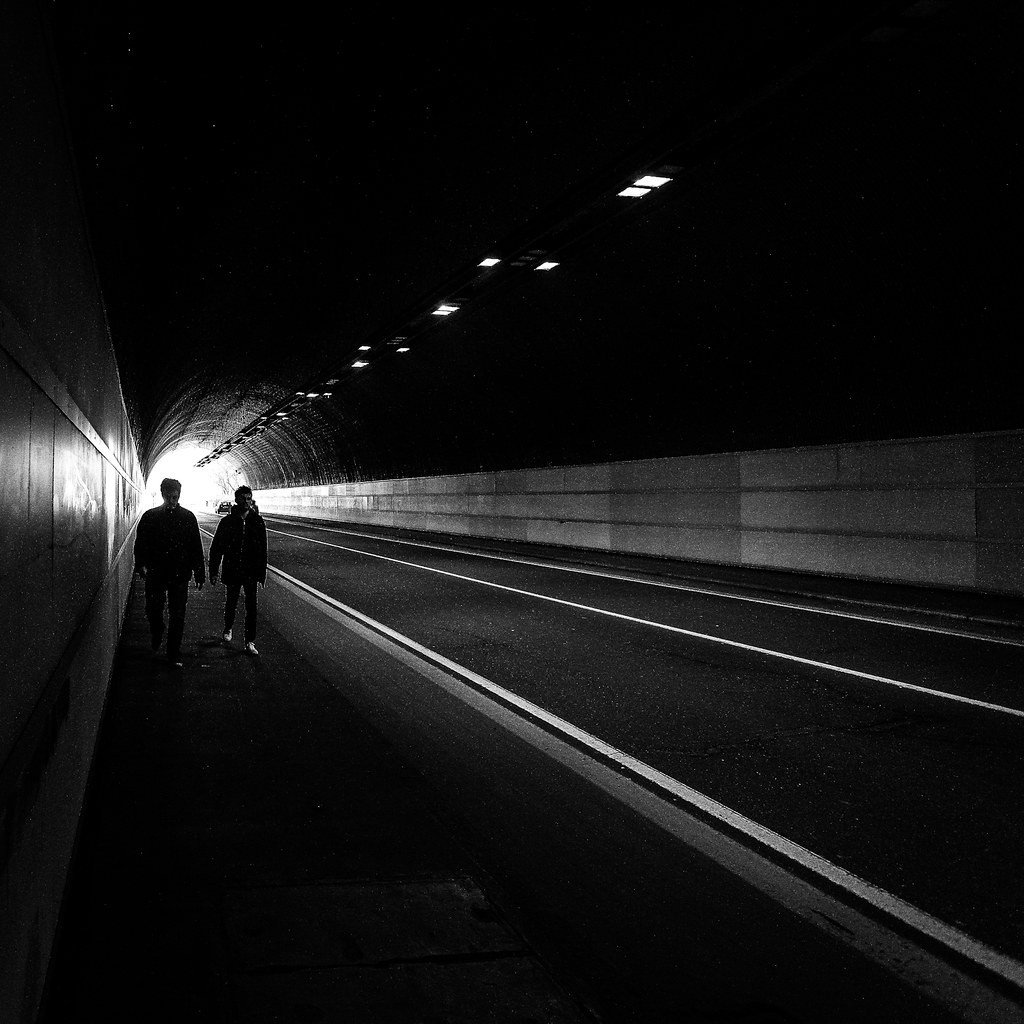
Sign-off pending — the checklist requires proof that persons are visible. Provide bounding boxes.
[133,478,206,669]
[250,499,259,515]
[208,485,268,656]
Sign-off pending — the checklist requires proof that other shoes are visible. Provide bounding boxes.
[246,642,258,655]
[152,634,163,649]
[223,629,233,641]
[168,652,183,668]
